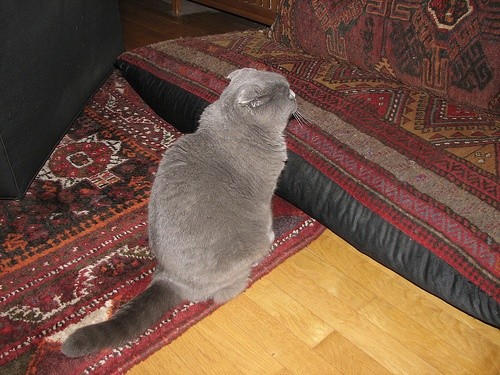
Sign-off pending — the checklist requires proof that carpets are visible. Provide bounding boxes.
[0,66,328,375]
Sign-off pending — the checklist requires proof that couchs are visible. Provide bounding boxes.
[115,0,500,325]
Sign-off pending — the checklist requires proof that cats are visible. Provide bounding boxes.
[59,68,315,360]
